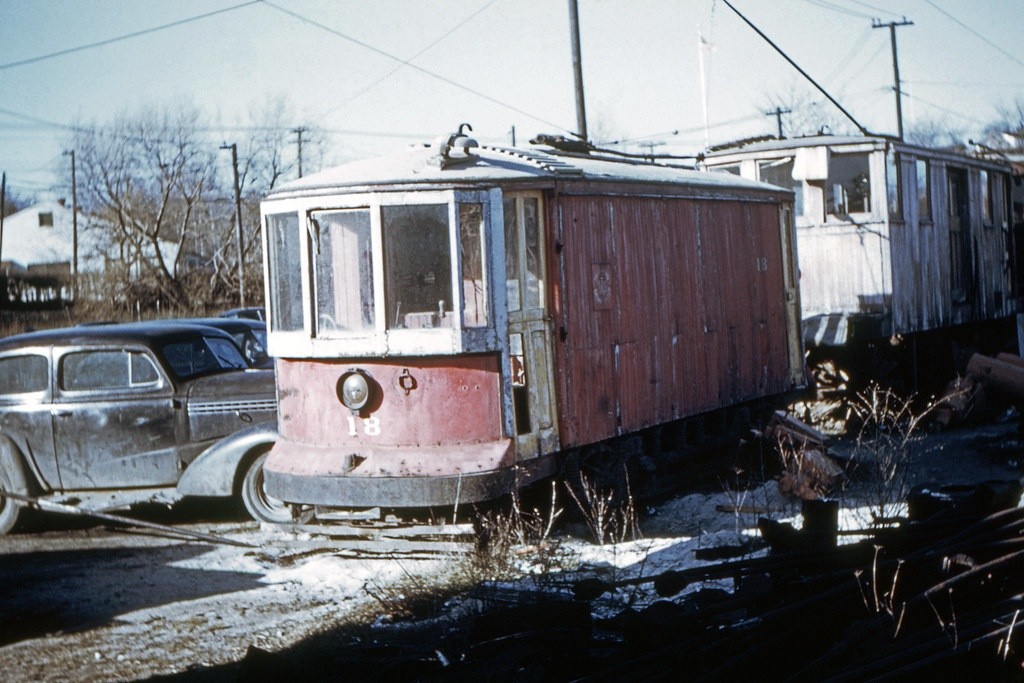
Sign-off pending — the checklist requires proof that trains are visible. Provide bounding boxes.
[262,120,1024,510]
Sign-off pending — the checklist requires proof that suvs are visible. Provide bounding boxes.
[0,316,297,537]
